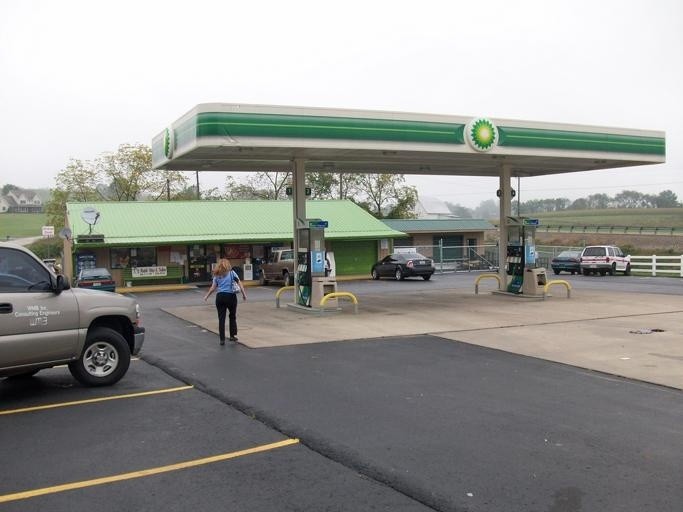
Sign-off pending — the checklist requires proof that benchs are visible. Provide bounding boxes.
[121,265,185,287]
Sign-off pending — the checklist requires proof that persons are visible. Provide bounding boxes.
[202,258,247,345]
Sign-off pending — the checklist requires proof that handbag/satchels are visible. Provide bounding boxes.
[232,277,241,294]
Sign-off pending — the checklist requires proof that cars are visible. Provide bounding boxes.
[0,242,145,387]
[370,253,435,281]
[551,245,631,276]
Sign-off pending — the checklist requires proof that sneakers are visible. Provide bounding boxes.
[219,336,239,345]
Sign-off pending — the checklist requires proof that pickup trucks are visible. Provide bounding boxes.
[258,249,294,286]
